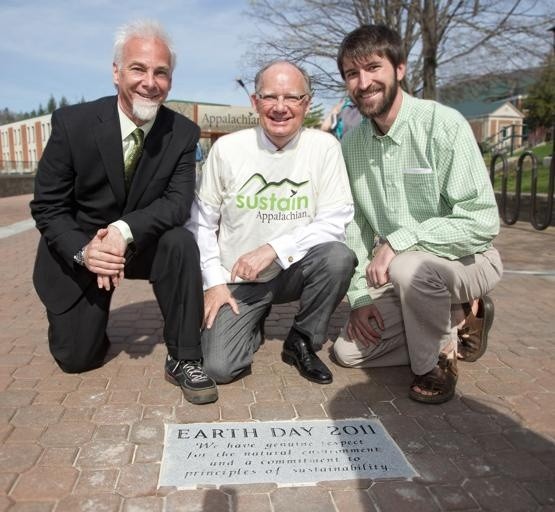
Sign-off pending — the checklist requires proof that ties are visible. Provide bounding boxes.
[122,127,144,196]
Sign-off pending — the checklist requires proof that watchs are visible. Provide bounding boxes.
[73,246,85,267]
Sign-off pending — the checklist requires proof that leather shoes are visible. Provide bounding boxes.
[281,326,332,383]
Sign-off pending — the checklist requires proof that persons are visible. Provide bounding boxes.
[340,24,502,405]
[28,17,218,406]
[189,58,359,385]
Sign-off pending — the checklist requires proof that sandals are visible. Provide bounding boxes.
[457,294,494,363]
[409,355,459,403]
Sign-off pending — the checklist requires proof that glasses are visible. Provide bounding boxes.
[258,93,307,105]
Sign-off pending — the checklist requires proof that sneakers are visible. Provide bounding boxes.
[165,353,218,405]
